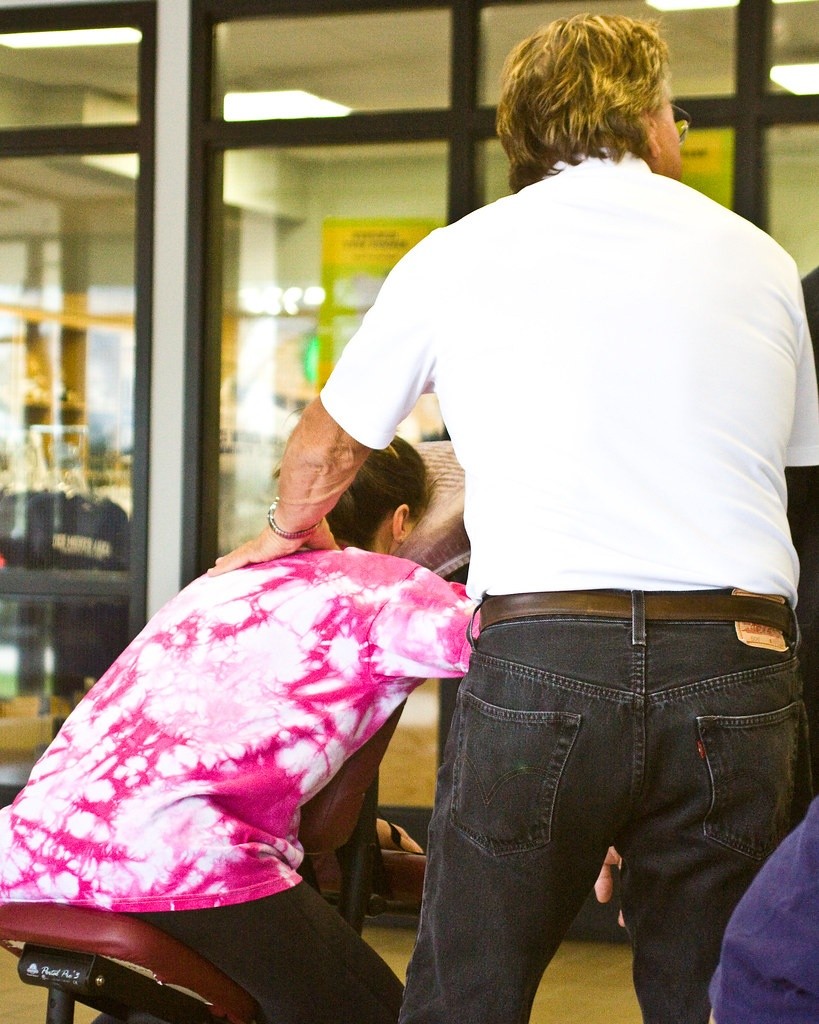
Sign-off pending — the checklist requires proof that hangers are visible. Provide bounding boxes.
[0,447,103,503]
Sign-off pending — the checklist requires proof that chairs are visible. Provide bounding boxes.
[0,441,470,1024]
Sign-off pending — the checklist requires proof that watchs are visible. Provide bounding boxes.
[267,494,323,541]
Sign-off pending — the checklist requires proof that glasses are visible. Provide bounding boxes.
[662,101,693,145]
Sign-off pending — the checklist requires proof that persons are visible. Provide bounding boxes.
[0,437,485,1024]
[202,11,816,1024]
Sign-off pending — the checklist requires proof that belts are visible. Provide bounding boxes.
[480,590,792,635]
